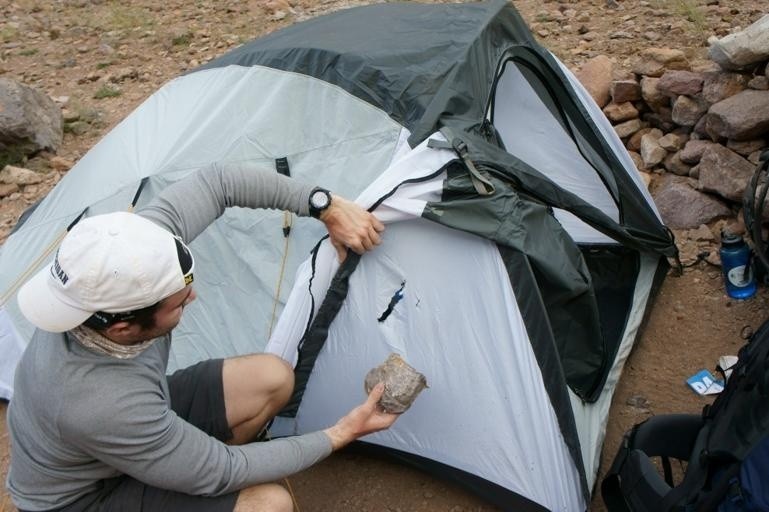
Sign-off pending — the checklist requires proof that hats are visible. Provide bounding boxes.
[17,213,194,333]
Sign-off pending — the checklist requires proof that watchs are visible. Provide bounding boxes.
[307,188,331,217]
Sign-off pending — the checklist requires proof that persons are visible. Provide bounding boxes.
[6,159,399,512]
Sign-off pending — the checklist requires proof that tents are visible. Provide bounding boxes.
[1,1,682,512]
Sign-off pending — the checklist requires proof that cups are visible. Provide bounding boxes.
[718,226,757,298]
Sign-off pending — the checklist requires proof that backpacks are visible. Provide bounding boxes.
[600,320,768,511]
[743,150,769,266]
